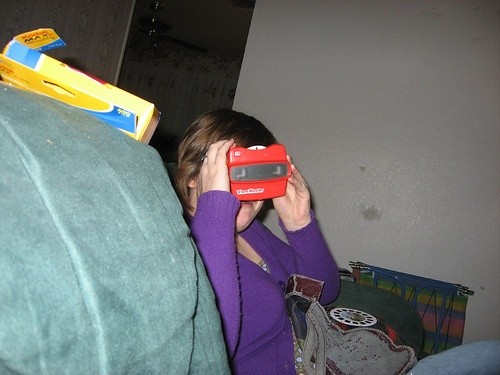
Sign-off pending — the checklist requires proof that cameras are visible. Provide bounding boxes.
[226,144,291,201]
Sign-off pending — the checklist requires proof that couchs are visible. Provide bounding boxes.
[1,82,424,375]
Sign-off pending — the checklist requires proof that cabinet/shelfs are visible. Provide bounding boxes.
[2,0,257,166]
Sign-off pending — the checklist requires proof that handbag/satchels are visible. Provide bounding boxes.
[286,273,423,372]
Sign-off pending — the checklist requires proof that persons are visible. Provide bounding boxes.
[172,109,340,374]
